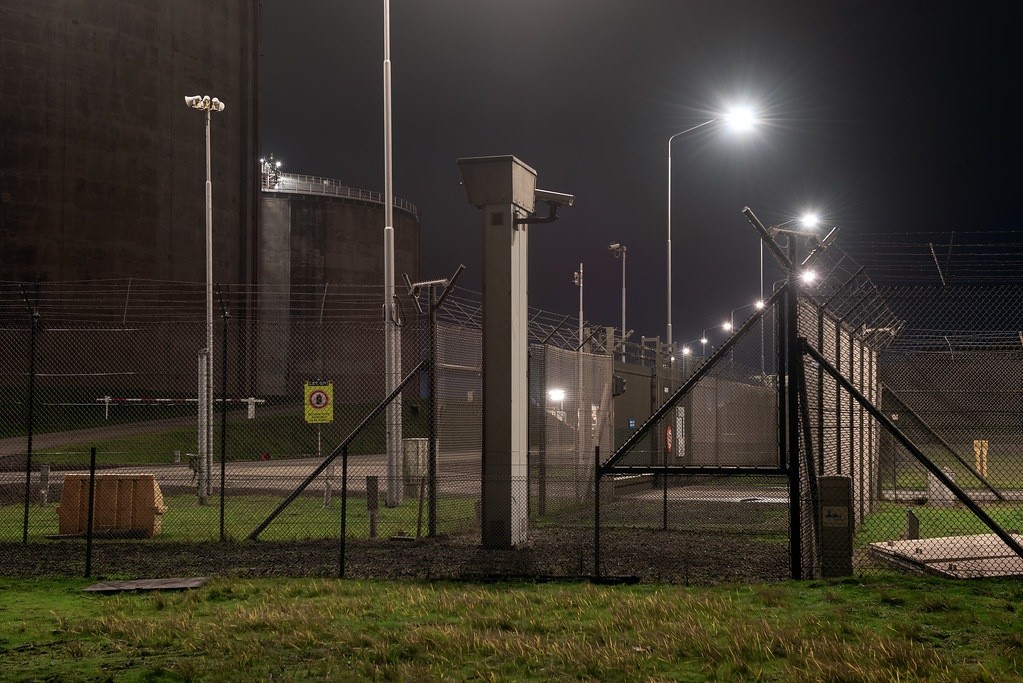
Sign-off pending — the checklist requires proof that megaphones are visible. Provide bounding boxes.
[184,95,225,112]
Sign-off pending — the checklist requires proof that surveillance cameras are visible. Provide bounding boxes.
[534,187,575,206]
[609,244,619,250]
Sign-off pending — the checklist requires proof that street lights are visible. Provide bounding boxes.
[667,107,753,362]
[548,389,564,449]
[731,301,763,373]
[682,338,707,381]
[761,214,817,372]
[702,321,729,366]
